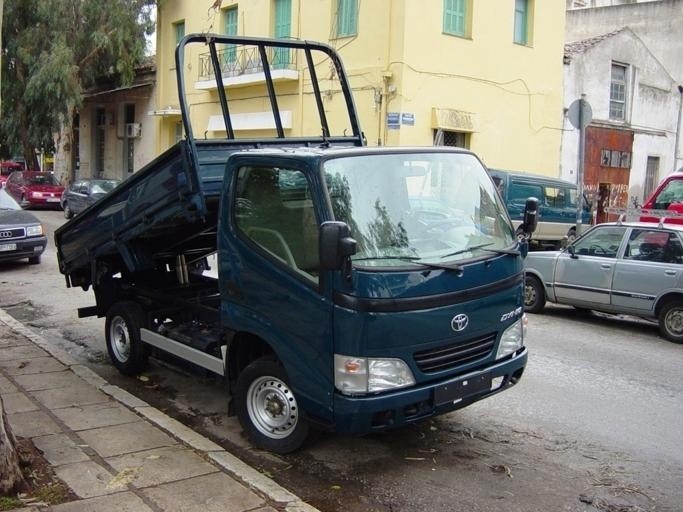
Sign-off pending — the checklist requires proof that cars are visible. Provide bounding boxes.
[0,187,48,265]
[60,178,123,220]
[523,221,683,343]
[0,160,65,211]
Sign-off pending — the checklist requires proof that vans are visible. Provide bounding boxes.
[485,167,595,248]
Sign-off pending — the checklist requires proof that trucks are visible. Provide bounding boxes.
[53,31,530,457]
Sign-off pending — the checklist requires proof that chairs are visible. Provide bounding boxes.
[239,171,320,268]
[628,243,664,259]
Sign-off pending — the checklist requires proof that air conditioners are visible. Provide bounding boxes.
[123,123,143,138]
